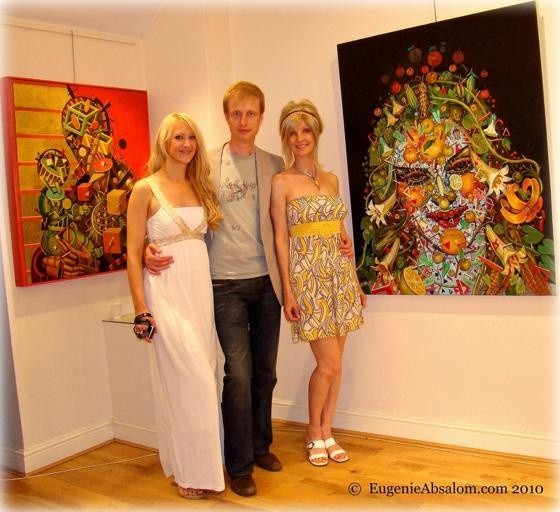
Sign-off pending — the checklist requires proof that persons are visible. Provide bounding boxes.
[270,98,366,467]
[127,112,225,499]
[144,82,353,498]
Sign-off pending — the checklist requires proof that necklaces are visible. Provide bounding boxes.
[293,162,321,190]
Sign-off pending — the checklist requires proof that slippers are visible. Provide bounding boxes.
[305,439,329,466]
[325,437,348,462]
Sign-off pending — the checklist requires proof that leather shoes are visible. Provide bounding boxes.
[255,452,282,471]
[230,474,256,494]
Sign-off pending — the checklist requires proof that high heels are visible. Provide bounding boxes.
[207,488,227,494]
[177,484,204,500]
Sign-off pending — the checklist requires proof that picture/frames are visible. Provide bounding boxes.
[1,75,151,288]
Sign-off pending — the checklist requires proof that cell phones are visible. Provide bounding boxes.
[146,324,156,341]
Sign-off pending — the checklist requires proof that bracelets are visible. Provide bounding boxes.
[134,313,153,319]
